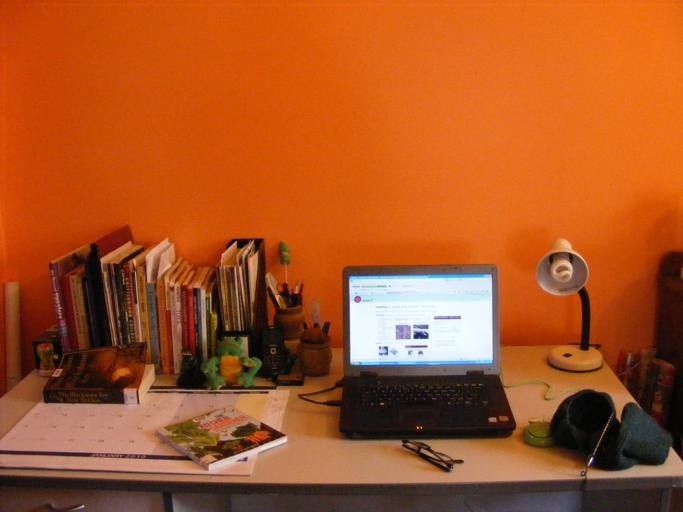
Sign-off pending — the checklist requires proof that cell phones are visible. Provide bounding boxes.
[261,325,286,376]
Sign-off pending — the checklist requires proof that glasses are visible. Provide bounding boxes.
[401,437,465,474]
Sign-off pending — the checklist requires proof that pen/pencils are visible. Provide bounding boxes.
[272,241,307,308]
[298,303,334,343]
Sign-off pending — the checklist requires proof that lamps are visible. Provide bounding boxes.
[534,239,603,373]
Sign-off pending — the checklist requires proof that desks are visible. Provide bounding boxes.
[0,344,683,512]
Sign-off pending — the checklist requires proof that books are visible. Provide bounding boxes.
[157,405,288,471]
[43,341,156,404]
[49,223,145,352]
[144,237,266,376]
[1,388,290,476]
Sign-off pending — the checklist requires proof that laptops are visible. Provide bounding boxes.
[339,264,516,440]
